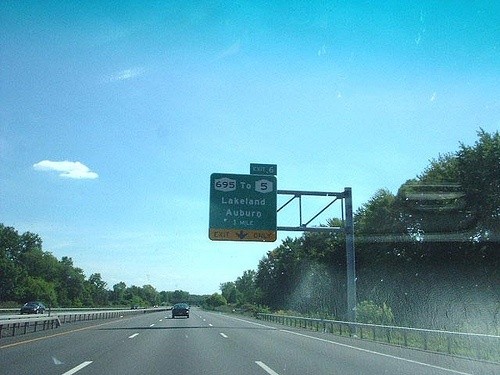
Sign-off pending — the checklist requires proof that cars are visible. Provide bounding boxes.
[20,302,45,314]
[130,304,146,313]
[170,303,190,319]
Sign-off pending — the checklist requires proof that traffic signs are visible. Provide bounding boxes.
[250,163,278,176]
[208,173,278,242]
